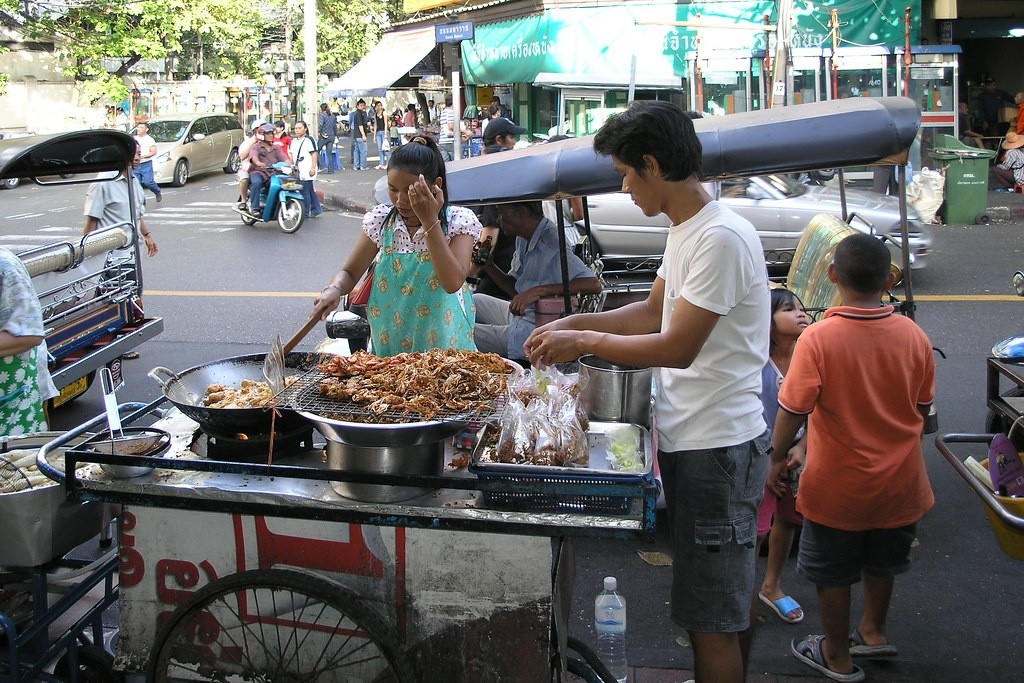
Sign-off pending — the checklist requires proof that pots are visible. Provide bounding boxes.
[148,352,345,433]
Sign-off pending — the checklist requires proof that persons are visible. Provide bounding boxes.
[100,105,127,132]
[541,135,584,251]
[0,247,59,439]
[238,120,322,217]
[439,96,509,162]
[153,124,167,139]
[83,140,158,359]
[755,288,809,623]
[958,78,1024,193]
[468,118,526,299]
[310,135,483,357]
[133,123,162,205]
[318,98,440,174]
[523,102,773,683]
[769,235,936,683]
[473,201,602,361]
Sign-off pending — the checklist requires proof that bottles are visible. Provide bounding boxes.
[477,235,493,265]
[595,576,627,683]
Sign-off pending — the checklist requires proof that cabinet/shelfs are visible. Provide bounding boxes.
[967,86,984,108]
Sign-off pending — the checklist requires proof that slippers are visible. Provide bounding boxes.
[759,591,804,623]
[791,635,865,683]
[994,188,1009,193]
[848,629,898,656]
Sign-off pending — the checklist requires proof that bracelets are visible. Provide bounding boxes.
[322,286,342,296]
[144,232,151,236]
[424,220,441,236]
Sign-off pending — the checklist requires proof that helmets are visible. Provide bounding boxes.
[256,125,273,141]
[251,119,267,131]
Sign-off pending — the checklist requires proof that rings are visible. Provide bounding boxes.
[411,192,417,196]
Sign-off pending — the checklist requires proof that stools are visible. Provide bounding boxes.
[1014,182,1024,196]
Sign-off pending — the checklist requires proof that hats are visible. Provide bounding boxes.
[1002,132,1024,149]
[490,96,499,102]
[483,117,526,142]
[984,77,994,83]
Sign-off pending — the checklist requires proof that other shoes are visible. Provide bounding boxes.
[381,165,388,170]
[156,190,161,202]
[249,207,260,216]
[374,164,381,169]
[361,167,369,170]
[354,167,359,171]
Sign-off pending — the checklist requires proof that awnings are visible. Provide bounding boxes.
[322,30,436,97]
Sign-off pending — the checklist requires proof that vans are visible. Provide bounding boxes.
[123,110,247,189]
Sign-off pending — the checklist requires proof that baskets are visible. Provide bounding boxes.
[467,464,633,517]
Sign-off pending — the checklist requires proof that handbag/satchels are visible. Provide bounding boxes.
[347,260,377,306]
[382,139,390,151]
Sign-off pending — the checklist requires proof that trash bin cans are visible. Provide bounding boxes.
[927,134,997,225]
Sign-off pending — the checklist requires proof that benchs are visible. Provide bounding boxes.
[768,212,903,325]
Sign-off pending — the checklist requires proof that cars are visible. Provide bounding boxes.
[564,170,933,291]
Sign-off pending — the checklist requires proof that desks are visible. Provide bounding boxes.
[971,136,1006,164]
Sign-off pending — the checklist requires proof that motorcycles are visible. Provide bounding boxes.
[0,125,167,418]
[315,92,940,473]
[232,150,309,235]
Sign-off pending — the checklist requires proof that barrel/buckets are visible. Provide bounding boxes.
[578,353,653,430]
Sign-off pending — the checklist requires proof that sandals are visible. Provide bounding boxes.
[238,203,246,210]
[122,351,139,359]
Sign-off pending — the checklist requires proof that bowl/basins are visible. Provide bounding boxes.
[324,436,445,504]
[92,441,171,477]
[290,354,526,446]
